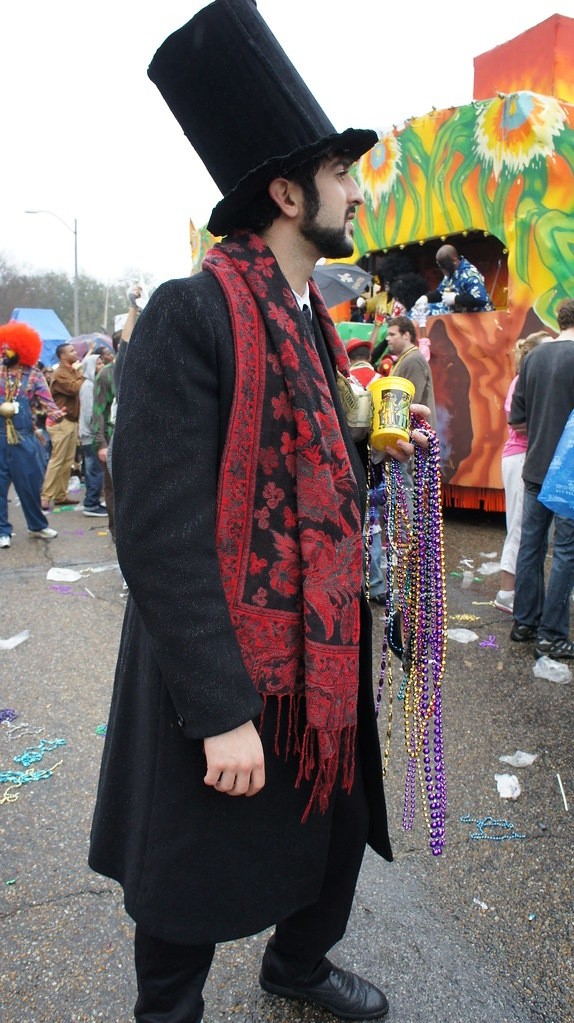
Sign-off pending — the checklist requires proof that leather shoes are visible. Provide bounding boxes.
[258,953,389,1019]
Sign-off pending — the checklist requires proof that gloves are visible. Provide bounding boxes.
[415,295,428,308]
[441,292,456,305]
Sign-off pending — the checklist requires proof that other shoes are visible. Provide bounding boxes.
[54,497,79,506]
[40,499,50,510]
[371,593,387,606]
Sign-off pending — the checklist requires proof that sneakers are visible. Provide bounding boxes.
[82,503,109,517]
[533,636,574,659]
[509,621,539,642]
[28,527,58,539]
[0,536,12,548]
[494,591,516,613]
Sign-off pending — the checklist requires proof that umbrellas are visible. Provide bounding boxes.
[311,263,373,311]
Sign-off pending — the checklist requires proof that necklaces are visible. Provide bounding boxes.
[388,346,418,376]
[362,410,447,857]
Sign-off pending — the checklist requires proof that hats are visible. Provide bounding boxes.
[147,0,378,238]
[346,338,372,355]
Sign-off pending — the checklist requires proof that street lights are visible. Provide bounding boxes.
[101,275,140,334]
[24,208,79,338]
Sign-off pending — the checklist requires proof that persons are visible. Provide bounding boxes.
[506,303,574,642]
[346,316,435,606]
[534,408,573,656]
[495,331,557,616]
[348,245,496,321]
[0,280,150,550]
[87,0,431,1023]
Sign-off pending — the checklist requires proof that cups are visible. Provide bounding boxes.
[368,376,415,452]
